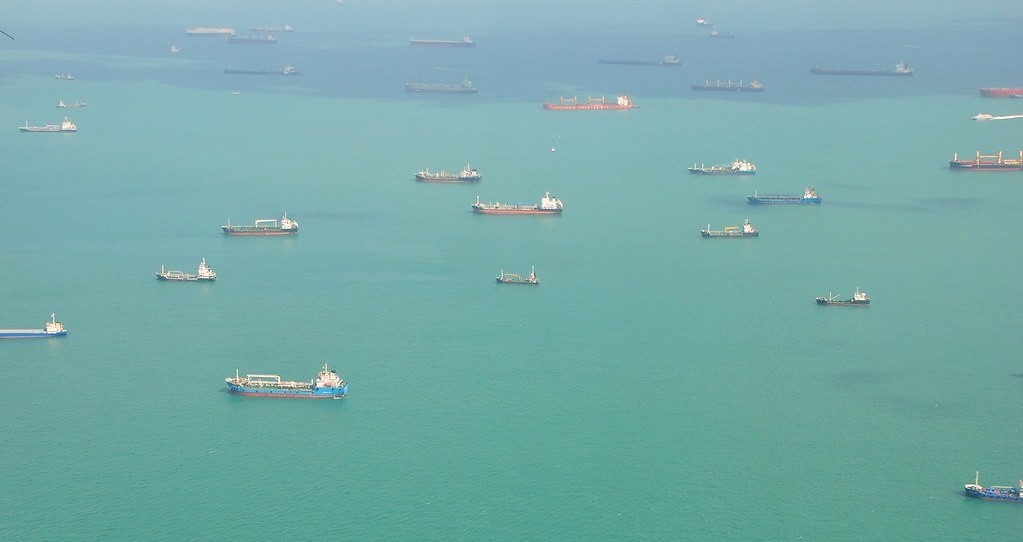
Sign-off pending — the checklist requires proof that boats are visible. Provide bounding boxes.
[18,116,77,133]
[414,162,482,183]
[224,362,348,400]
[686,158,756,176]
[470,191,564,215]
[0,312,68,339]
[55,72,74,80]
[700,218,760,237]
[56,98,86,108]
[965,470,1023,503]
[815,286,871,307]
[496,264,539,285]
[979,87,1023,98]
[971,112,1023,121]
[184,17,765,112]
[746,186,821,206]
[810,61,913,77]
[949,150,1023,172]
[156,257,217,281]
[221,211,299,237]
